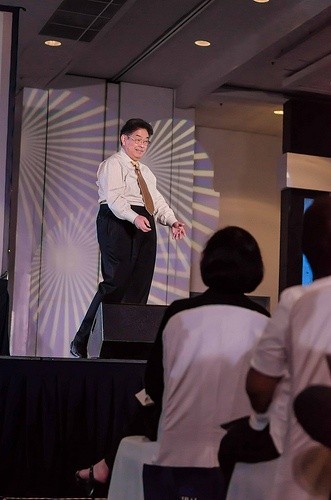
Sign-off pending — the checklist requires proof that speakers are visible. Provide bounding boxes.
[86,301,169,360]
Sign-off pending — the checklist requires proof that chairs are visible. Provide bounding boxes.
[106,277,331,500]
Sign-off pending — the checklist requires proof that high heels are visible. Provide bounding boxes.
[75,466,107,498]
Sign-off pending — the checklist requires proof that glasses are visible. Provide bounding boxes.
[128,135,150,146]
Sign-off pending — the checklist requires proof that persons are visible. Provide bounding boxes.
[218,192,331,500]
[70,119,186,357]
[67,225,271,498]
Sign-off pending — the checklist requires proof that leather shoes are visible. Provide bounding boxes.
[70,340,88,359]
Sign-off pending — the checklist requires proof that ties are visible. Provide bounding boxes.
[131,161,154,217]
